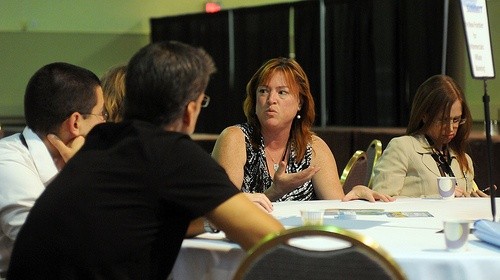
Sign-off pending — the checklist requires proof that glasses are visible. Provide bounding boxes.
[195,94,210,107]
[433,116,466,127]
[81,112,108,120]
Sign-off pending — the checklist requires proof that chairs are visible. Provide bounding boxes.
[366,140,383,187]
[341,148,367,194]
[232,224,409,280]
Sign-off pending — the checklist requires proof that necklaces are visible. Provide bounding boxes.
[266,150,284,171]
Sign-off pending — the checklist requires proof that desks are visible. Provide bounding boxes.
[168,193,500,280]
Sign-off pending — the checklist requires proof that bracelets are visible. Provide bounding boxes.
[202,218,220,234]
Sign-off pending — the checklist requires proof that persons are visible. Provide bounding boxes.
[371,75,490,199]
[100,67,127,122]
[7,41,285,280]
[211,58,396,204]
[0,62,108,280]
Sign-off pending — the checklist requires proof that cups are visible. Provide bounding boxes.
[301,210,324,225]
[436,177,456,199]
[443,221,469,253]
